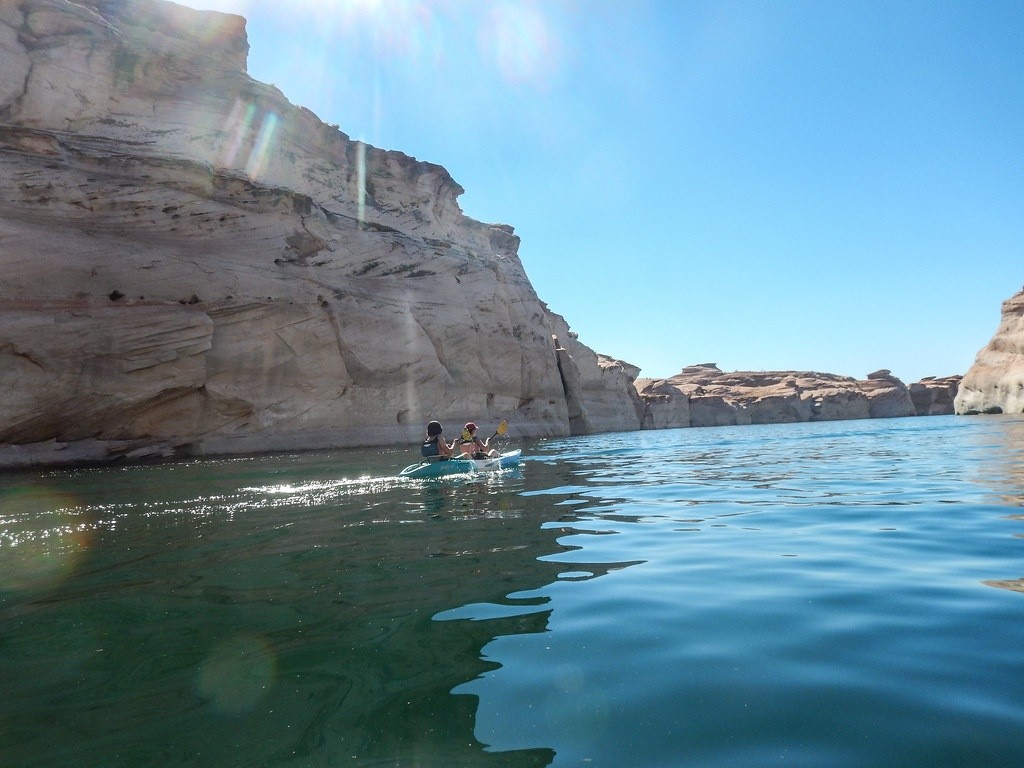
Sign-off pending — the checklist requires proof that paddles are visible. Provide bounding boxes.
[489,418,508,442]
[450,426,473,447]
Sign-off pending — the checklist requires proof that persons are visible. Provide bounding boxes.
[458,423,503,460]
[421,421,470,460]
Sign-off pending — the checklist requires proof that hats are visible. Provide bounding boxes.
[466,423,478,430]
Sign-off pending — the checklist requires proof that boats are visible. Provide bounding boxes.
[397,448,523,480]
